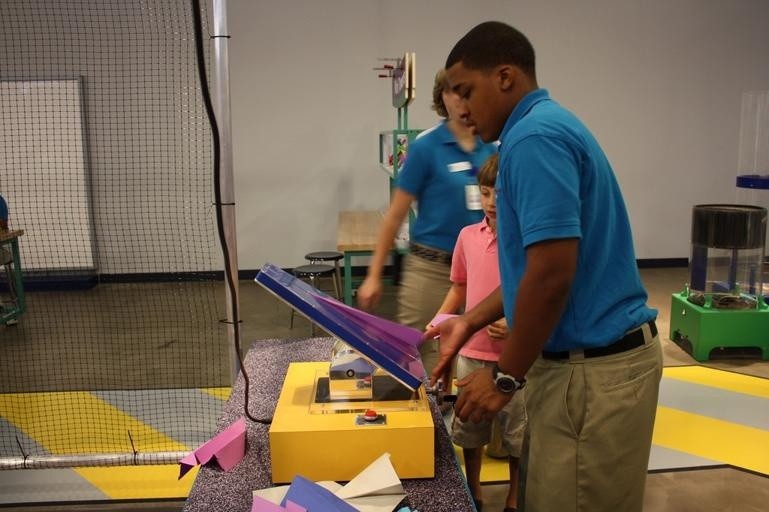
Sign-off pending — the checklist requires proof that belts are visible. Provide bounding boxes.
[410,244,454,264]
[543,322,658,362]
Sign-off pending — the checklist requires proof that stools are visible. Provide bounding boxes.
[287,250,345,339]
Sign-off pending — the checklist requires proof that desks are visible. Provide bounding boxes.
[0,228,29,326]
[336,208,410,307]
[183,333,476,511]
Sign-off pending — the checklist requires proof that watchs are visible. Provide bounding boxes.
[492,363,527,394]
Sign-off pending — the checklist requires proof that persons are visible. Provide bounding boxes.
[425,150,528,512]
[416,21,663,512]
[357,66,499,378]
